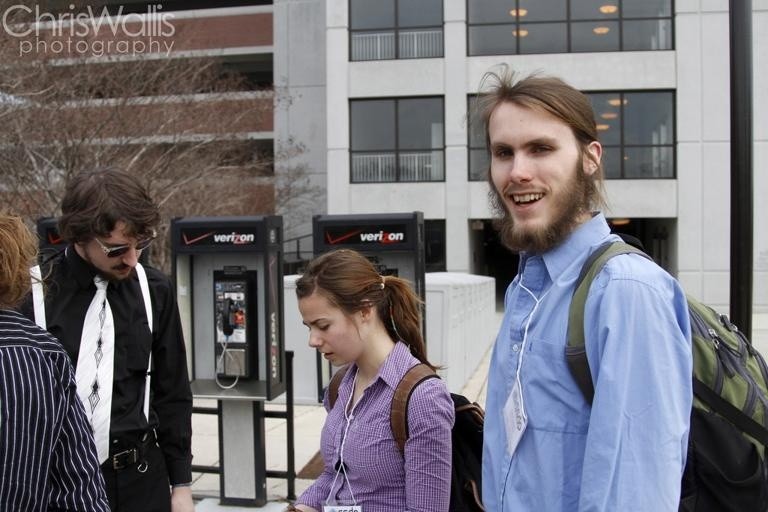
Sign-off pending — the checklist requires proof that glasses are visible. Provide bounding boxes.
[93,228,157,257]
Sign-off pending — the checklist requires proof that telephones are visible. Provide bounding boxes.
[222,299,235,336]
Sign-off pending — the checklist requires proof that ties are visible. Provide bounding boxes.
[74,275,115,466]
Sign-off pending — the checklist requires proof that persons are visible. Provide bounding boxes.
[481,62,695,511]
[13,165,194,512]
[280,247,459,512]
[1,210,114,511]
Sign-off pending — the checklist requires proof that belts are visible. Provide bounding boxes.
[101,432,153,473]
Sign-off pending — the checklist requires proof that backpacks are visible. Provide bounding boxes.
[564,239,768,512]
[329,363,484,512]
